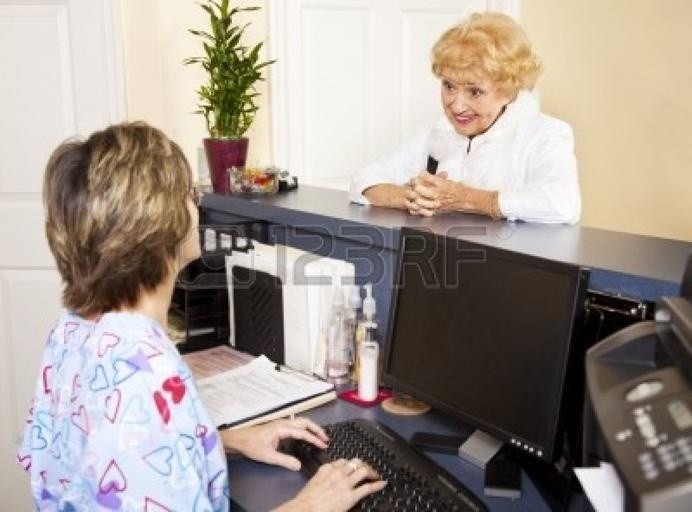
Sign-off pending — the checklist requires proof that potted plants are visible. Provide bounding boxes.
[183,0,281,192]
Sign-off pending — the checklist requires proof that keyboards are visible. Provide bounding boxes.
[289,418,493,512]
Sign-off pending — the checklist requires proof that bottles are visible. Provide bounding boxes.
[323,280,381,402]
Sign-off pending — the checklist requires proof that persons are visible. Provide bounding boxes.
[349,6,585,225]
[11,114,388,512]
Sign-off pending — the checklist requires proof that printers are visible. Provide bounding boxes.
[582,295,692,512]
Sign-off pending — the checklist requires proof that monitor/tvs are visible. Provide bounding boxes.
[380,225,591,500]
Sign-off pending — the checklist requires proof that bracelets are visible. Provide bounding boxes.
[488,190,500,221]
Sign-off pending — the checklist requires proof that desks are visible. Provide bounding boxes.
[224,383,552,511]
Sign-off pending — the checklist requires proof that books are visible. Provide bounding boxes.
[193,354,337,431]
[222,243,356,380]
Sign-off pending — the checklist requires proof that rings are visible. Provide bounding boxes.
[346,460,357,470]
